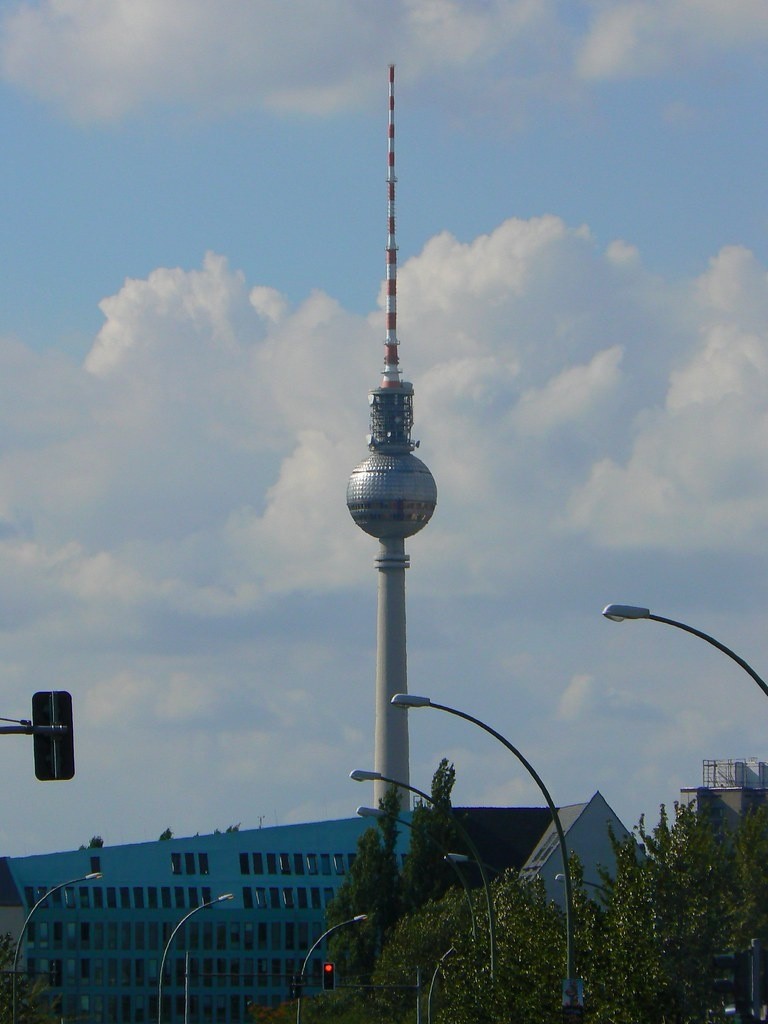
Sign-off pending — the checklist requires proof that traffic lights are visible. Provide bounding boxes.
[708,950,752,1021]
[323,962,336,991]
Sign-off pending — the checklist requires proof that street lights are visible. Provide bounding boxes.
[425,947,460,1024]
[10,872,104,1024]
[156,894,236,1024]
[388,693,576,984]
[296,913,368,1024]
[355,806,483,970]
[351,769,499,993]
